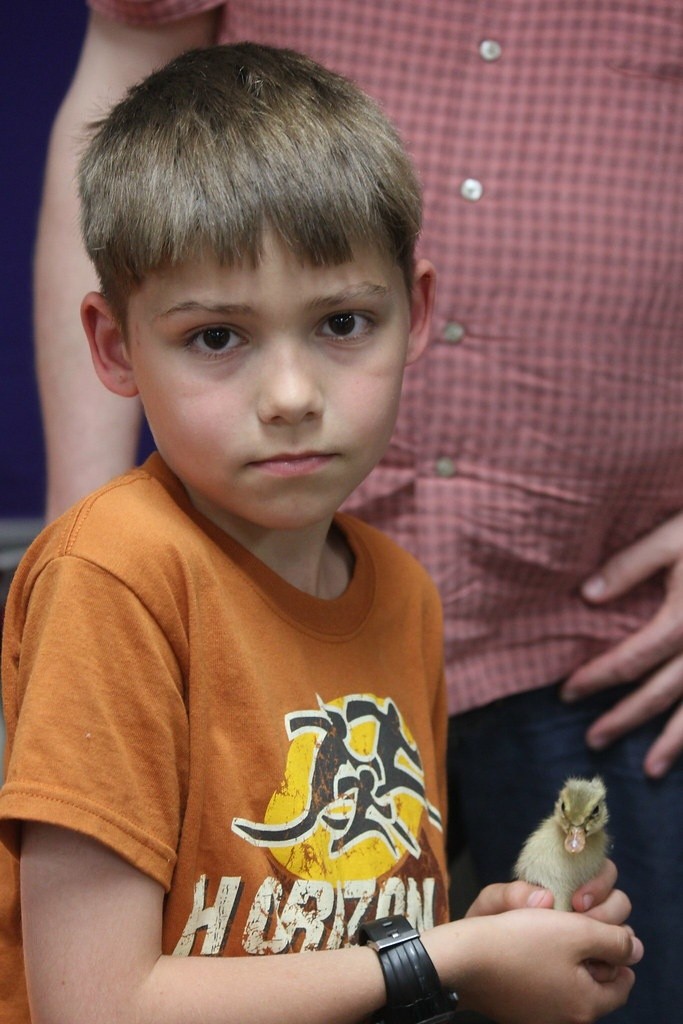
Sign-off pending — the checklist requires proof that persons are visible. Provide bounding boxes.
[0,41,643,1024]
[32,0,682,1024]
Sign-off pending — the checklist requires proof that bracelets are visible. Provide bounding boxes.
[357,914,459,1024]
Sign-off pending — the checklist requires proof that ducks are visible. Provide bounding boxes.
[510,780,609,911]
